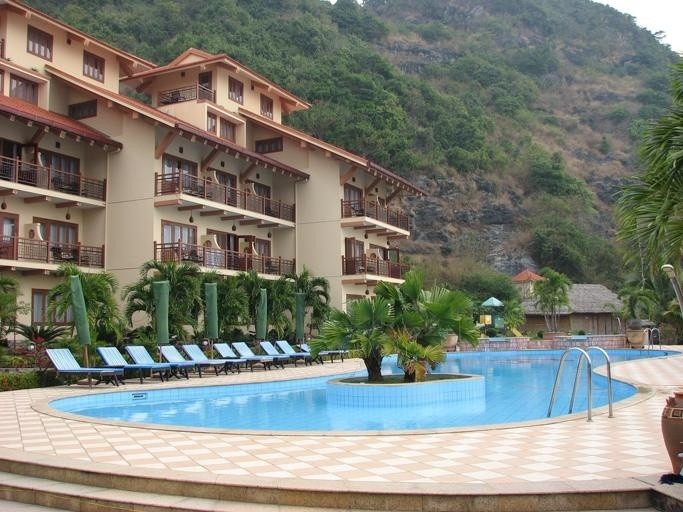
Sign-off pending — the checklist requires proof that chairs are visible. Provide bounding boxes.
[157,344,225,377]
[260,342,315,367]
[174,175,278,216]
[183,342,246,375]
[99,346,171,384]
[49,242,92,267]
[231,342,290,371]
[296,344,349,363]
[276,340,328,365]
[213,343,273,371]
[43,349,126,387]
[125,344,195,380]
[172,244,282,276]
[48,174,92,197]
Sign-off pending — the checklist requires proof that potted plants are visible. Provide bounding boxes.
[624,318,649,351]
[529,265,572,339]
[307,270,487,405]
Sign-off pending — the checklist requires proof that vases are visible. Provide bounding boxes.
[661,390,683,475]
